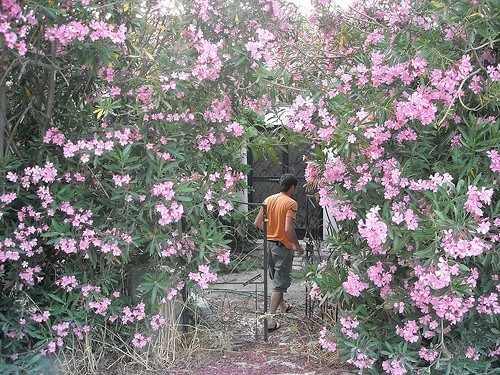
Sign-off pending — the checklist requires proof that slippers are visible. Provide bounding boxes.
[266,321,281,333]
[285,303,294,312]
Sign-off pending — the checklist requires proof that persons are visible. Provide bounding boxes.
[253,173,304,333]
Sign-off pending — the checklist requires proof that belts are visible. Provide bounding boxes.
[267,240,283,246]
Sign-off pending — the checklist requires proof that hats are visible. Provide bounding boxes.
[279,174,299,192]
